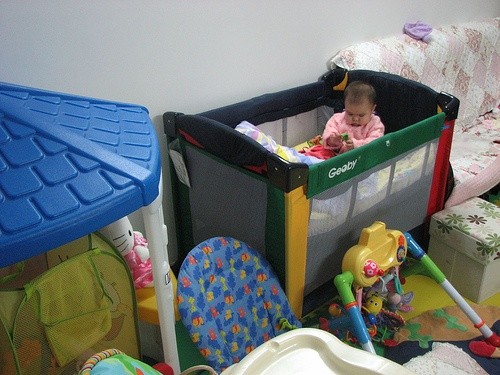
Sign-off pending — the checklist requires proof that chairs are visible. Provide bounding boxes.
[177,236,305,375]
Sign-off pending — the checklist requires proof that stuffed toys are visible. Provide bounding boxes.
[365,295,383,319]
[96,215,153,290]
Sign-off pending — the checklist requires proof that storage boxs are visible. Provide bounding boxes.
[428,195,500,303]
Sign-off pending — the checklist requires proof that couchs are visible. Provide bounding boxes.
[330,16,499,209]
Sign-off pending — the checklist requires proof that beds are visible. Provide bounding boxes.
[163,65,461,321]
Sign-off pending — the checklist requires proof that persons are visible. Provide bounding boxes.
[320,80,385,154]
